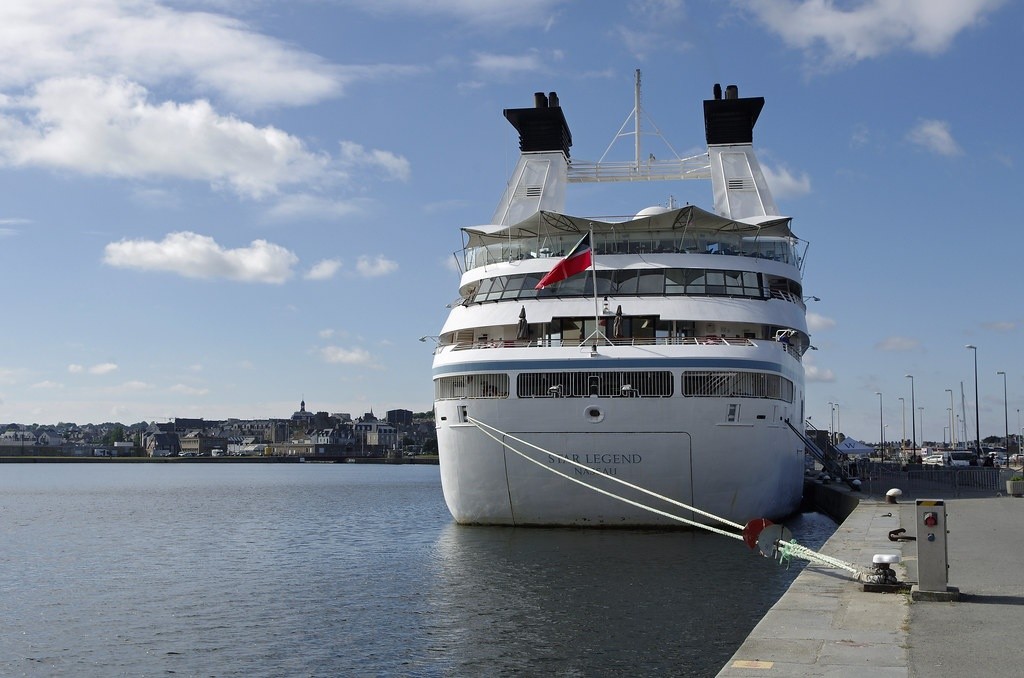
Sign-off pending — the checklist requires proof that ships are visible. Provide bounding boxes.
[417,69,819,528]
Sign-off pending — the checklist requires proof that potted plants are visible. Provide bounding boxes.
[1006,476,1024,496]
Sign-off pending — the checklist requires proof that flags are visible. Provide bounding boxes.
[535,229,594,290]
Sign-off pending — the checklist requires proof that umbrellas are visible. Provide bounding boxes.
[613,305,624,339]
[516,305,529,340]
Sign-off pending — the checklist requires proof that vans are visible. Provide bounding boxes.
[923,454,944,464]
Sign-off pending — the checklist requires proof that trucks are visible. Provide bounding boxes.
[211,449,225,457]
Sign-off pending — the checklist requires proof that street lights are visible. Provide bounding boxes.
[996,371,1010,467]
[827,401,833,445]
[869,392,888,452]
[1016,409,1021,455]
[918,407,925,447]
[834,403,840,433]
[965,344,979,458]
[898,397,906,456]
[905,374,916,463]
[946,408,952,449]
[944,389,955,450]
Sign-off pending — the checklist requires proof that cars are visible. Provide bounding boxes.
[165,452,177,457]
[178,451,197,458]
[197,452,210,457]
